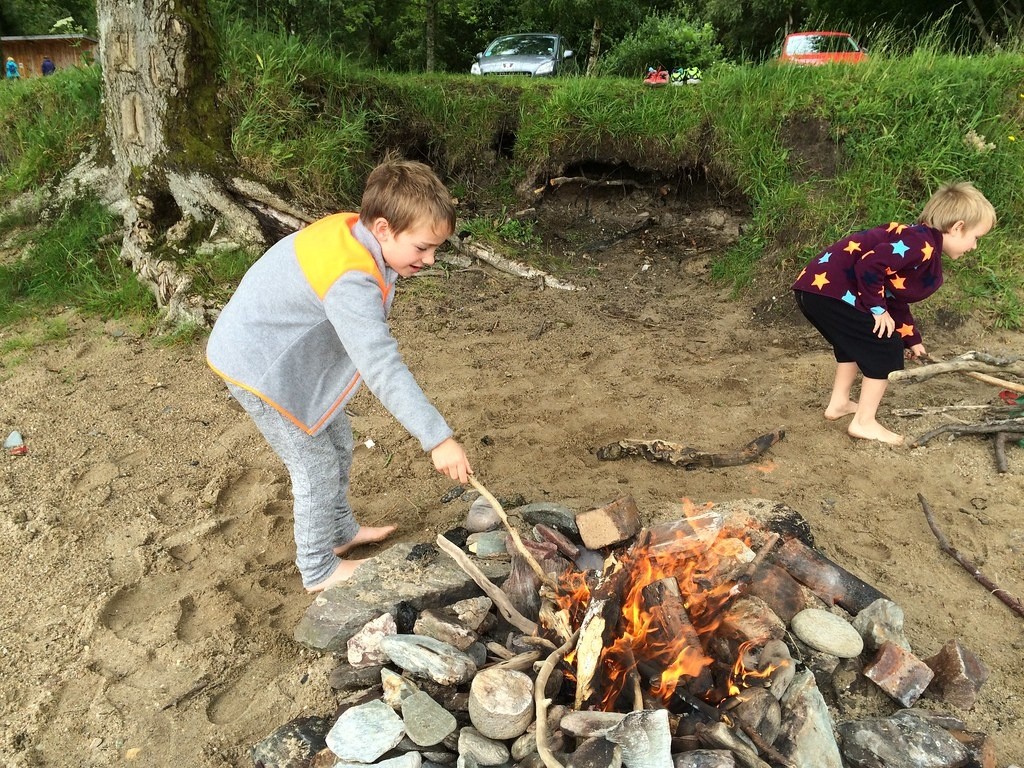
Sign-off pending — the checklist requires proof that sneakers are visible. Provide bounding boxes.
[643,71,658,85]
[670,67,685,86]
[686,67,703,84]
[656,71,669,86]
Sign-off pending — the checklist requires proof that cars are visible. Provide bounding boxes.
[470,33,582,75]
[777,31,870,64]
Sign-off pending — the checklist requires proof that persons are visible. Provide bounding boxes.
[40,56,55,76]
[790,182,996,446]
[205,159,474,594]
[5,56,29,80]
